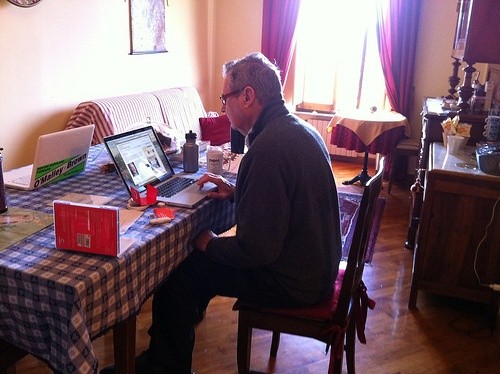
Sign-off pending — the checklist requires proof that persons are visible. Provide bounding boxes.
[135,53,342,374]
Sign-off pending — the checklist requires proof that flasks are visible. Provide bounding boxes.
[182,130,199,173]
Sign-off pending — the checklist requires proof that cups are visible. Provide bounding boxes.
[483,115,500,142]
[457,134,470,147]
[442,132,455,147]
[206,149,231,175]
[446,136,464,155]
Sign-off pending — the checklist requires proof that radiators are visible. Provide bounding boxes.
[307,119,357,157]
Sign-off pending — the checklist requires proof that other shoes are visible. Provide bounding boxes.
[99,349,162,374]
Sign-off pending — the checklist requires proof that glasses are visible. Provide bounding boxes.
[219,90,239,105]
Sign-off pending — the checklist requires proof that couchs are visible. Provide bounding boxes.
[65,83,250,169]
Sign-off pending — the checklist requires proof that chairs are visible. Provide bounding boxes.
[237,156,386,374]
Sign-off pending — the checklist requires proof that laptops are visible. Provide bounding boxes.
[103,125,219,209]
[3,124,95,190]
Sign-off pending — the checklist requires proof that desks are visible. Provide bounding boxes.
[326,108,411,184]
[408,141,500,310]
[0,141,236,374]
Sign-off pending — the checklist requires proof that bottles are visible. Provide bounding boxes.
[0,148,8,213]
[471,80,486,113]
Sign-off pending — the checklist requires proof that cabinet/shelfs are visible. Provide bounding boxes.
[450,0,500,63]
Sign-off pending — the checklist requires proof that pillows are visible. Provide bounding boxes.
[200,114,232,145]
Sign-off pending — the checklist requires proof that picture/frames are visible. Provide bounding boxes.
[128,1,167,54]
